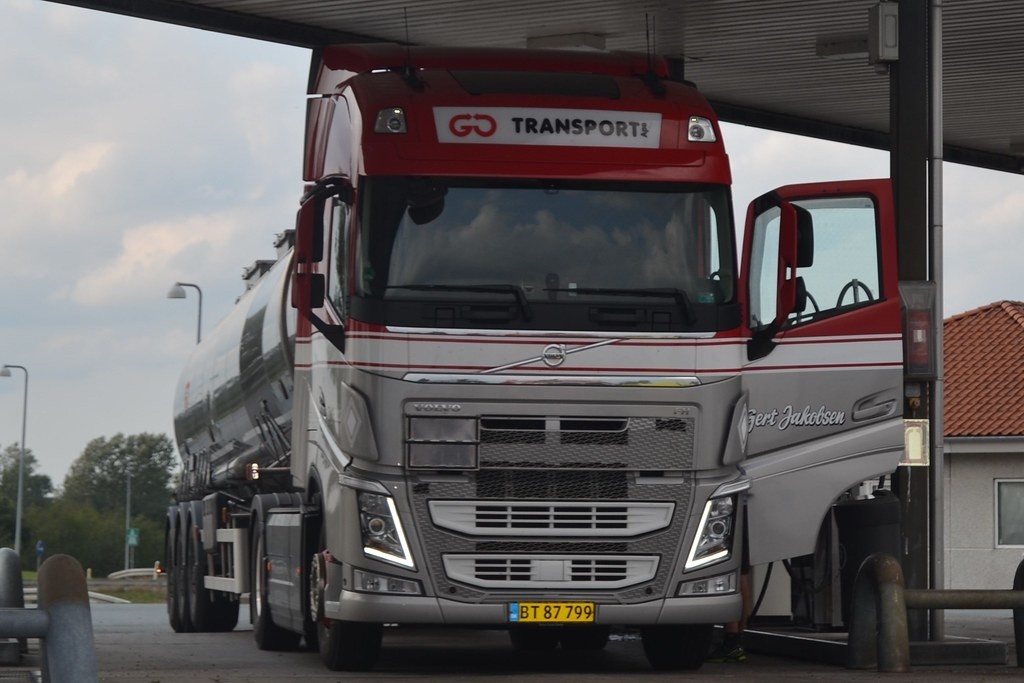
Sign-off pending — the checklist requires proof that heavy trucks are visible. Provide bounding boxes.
[163,40,906,669]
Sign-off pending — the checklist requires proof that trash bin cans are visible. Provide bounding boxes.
[832,487,902,633]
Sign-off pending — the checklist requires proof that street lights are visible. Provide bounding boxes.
[166,280,203,344]
[0,363,29,554]
